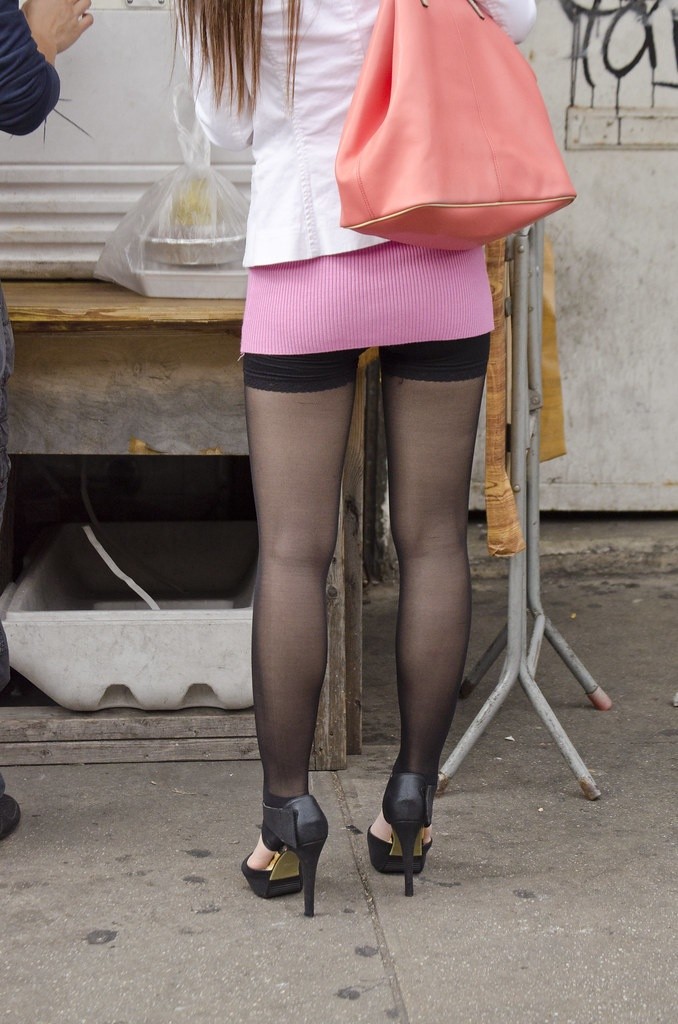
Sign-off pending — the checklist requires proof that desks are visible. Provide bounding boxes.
[0,282,378,772]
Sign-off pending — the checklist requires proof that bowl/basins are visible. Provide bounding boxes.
[136,234,247,265]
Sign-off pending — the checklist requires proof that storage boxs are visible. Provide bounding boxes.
[0,519,253,713]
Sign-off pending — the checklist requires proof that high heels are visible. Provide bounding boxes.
[366,772,436,898]
[241,795,328,916]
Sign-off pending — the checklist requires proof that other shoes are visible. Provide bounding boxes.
[1,794,20,841]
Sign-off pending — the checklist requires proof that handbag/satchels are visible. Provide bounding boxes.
[94,84,251,300]
[335,0,576,251]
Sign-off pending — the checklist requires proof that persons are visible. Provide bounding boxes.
[0,1,97,840]
[172,1,542,916]
